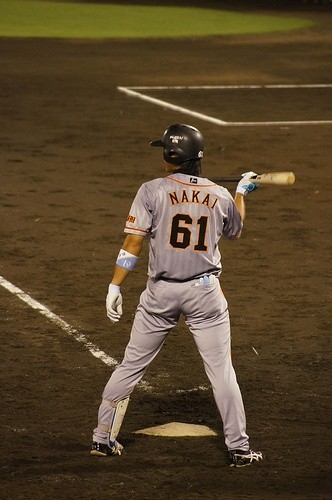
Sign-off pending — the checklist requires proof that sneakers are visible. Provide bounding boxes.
[229,449,264,467]
[90,441,127,456]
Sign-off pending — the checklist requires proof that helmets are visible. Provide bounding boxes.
[150,123,204,164]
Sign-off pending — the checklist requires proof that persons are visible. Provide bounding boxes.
[91,124,265,466]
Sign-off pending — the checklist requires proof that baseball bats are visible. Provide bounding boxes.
[207,171,296,188]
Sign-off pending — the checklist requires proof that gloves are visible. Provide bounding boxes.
[105,283,123,324]
[235,171,259,197]
